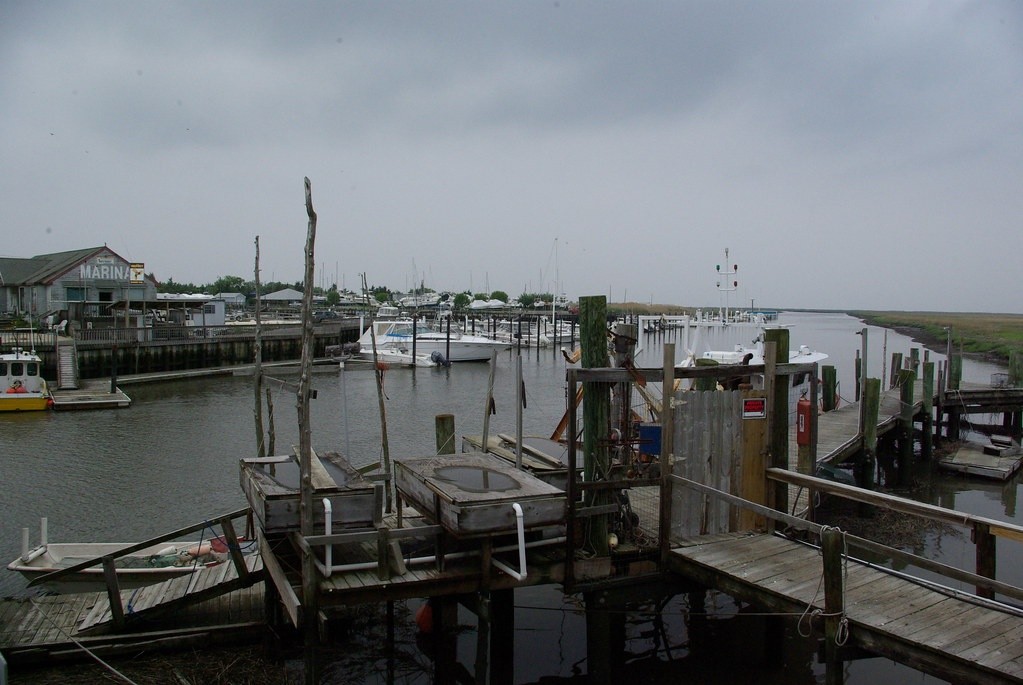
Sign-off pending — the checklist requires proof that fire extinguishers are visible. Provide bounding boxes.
[797,387,810,446]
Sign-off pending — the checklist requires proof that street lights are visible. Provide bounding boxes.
[944,327,951,392]
[751,297,755,313]
[854,326,868,435]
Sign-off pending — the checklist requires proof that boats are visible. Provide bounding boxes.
[7,518,259,594]
[940,431,1023,481]
[672,332,840,427]
[357,236,766,368]
[0,303,53,411]
